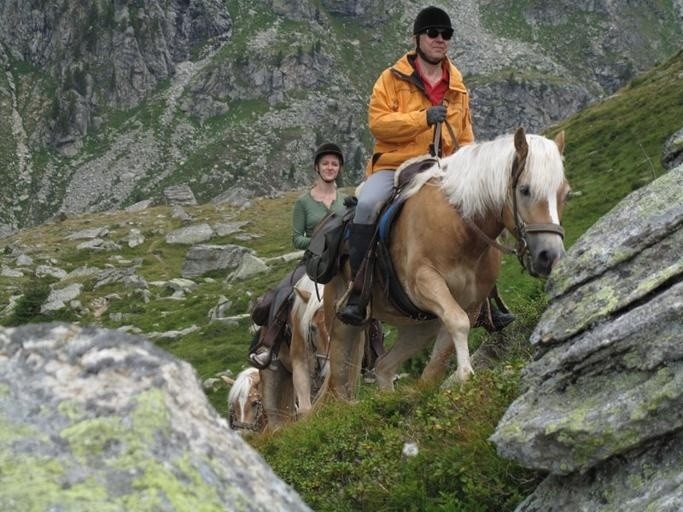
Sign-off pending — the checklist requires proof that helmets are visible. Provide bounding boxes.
[413,6,454,35]
[314,143,343,166]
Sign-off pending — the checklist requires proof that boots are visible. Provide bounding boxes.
[250,285,292,372]
[336,223,376,326]
[478,298,517,331]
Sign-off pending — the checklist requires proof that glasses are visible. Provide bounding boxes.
[420,29,453,41]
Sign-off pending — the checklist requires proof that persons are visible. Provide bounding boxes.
[336,7,515,331]
[249,143,353,369]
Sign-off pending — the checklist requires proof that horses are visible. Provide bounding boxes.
[323,128,570,396]
[220,366,261,440]
[258,272,333,429]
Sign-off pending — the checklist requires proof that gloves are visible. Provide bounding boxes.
[426,105,447,125]
